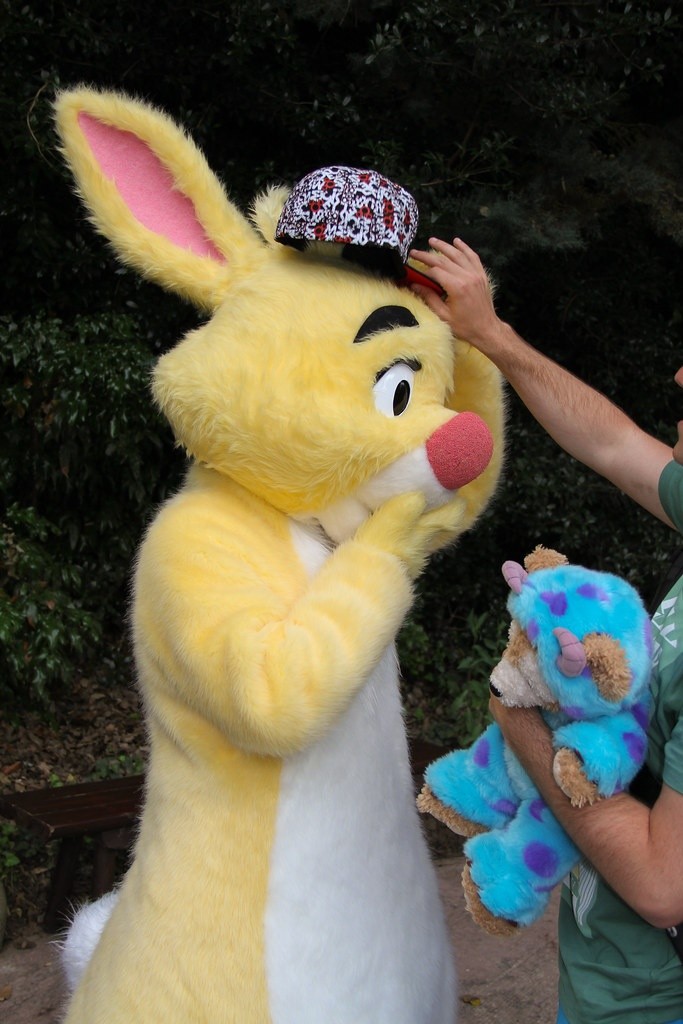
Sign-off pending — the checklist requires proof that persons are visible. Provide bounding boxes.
[402,236,683,1024]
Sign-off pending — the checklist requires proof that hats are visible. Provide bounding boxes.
[275,166,445,297]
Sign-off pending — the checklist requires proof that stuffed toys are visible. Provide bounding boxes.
[48,88,510,1024]
[417,548,651,933]
